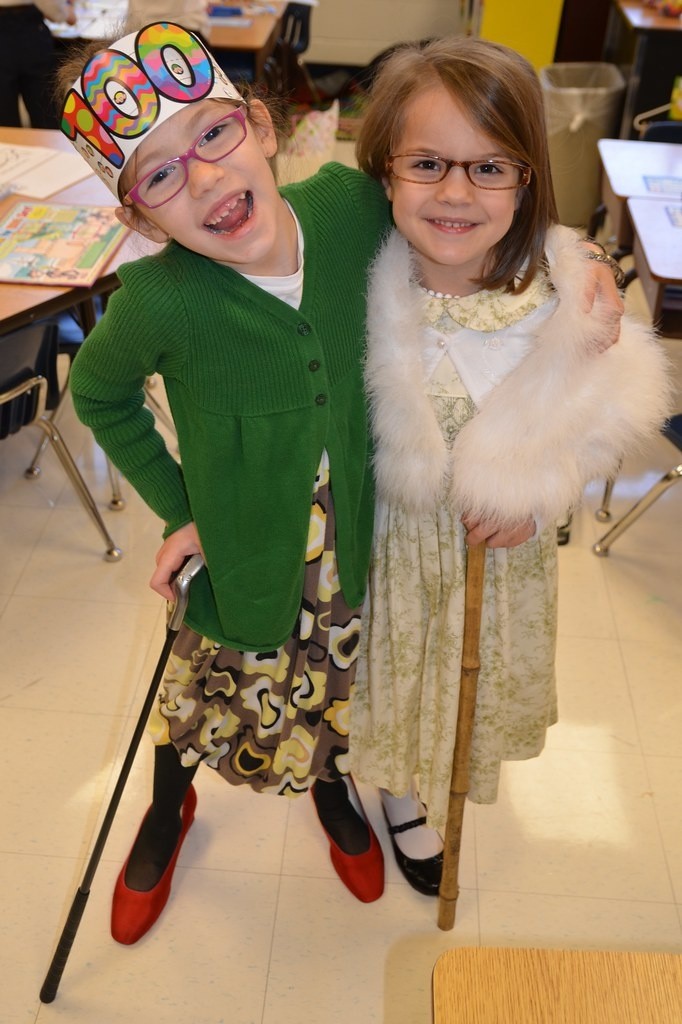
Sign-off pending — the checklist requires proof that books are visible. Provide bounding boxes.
[0,143,98,200]
[0,202,132,286]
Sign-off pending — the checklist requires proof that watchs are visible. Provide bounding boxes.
[574,236,607,255]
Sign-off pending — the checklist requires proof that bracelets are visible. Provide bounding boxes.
[576,250,625,288]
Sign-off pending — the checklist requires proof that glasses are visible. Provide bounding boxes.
[120,103,247,209]
[384,153,533,191]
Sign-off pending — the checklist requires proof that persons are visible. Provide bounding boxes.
[55,22,625,945]
[0,0,129,129]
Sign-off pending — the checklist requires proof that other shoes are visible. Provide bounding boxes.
[309,773,384,904]
[382,791,444,896]
[110,783,197,945]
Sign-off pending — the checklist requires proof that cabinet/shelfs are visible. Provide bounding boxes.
[457,1,562,73]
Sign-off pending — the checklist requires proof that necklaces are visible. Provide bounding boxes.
[422,287,461,301]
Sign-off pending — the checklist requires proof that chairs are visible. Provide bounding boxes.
[265,3,313,100]
[591,410,682,557]
[1,318,124,563]
[25,273,180,512]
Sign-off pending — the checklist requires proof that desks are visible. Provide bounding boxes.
[618,1,682,139]
[1,126,169,337]
[617,198,682,340]
[45,0,127,47]
[587,138,682,262]
[203,0,288,86]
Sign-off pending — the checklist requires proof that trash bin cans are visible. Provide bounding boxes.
[536,62,625,228]
